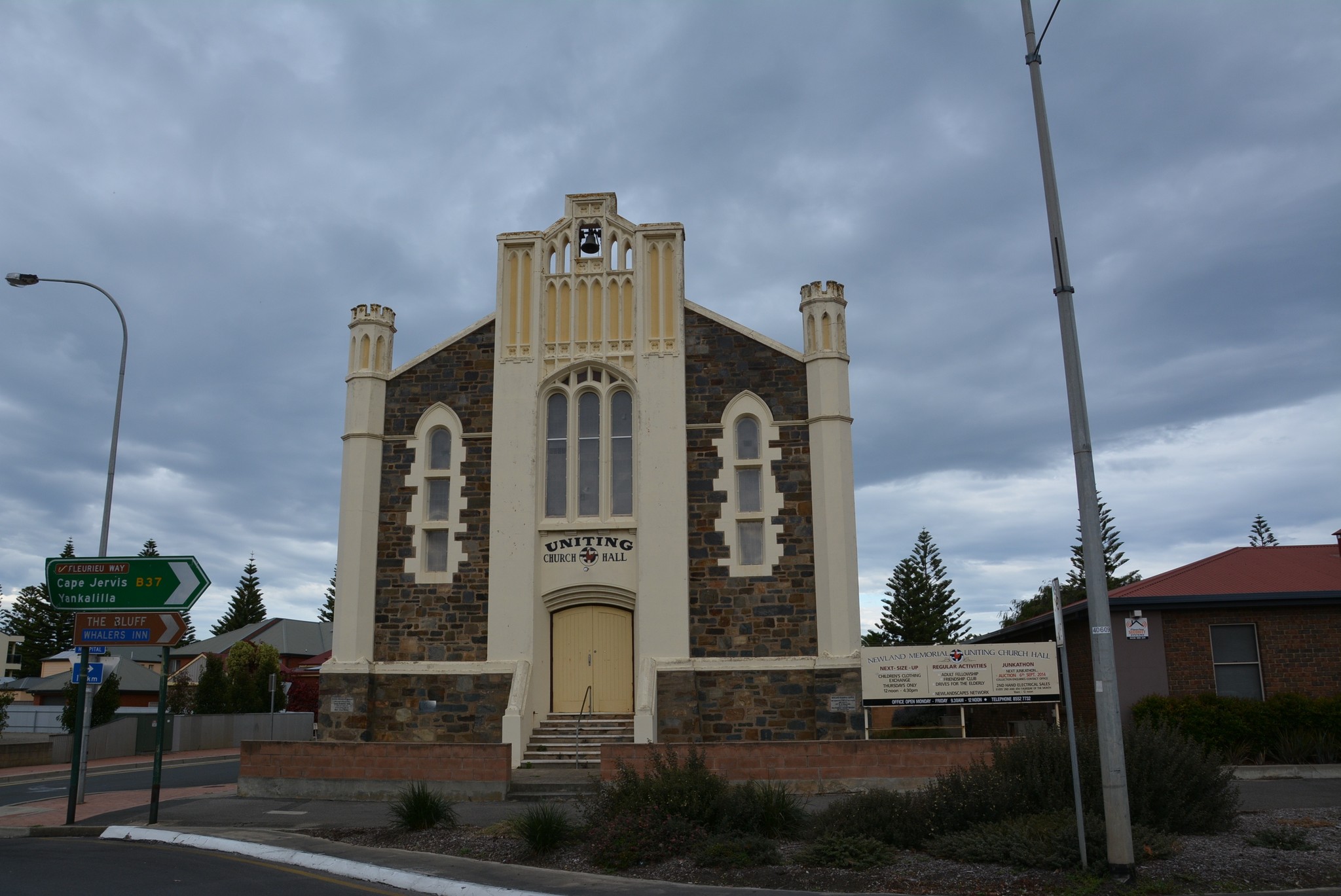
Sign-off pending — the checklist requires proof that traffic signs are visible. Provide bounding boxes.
[71,662,105,683]
[45,556,212,613]
[73,612,189,647]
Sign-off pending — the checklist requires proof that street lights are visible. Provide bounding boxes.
[4,271,130,822]
